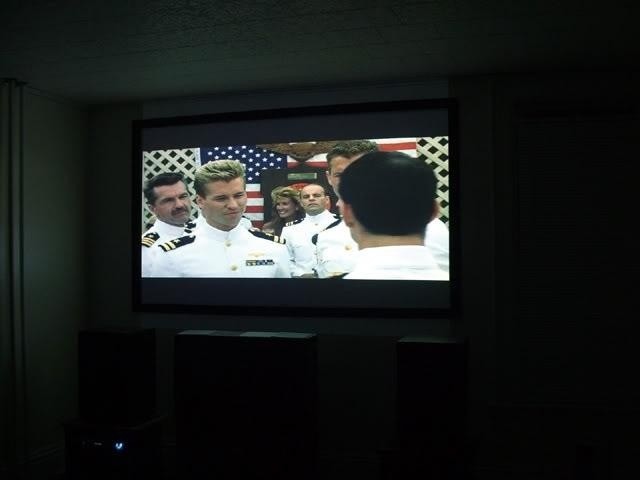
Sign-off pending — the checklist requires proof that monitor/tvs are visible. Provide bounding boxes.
[129,95,463,320]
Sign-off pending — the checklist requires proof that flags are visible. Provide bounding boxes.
[195,134,417,229]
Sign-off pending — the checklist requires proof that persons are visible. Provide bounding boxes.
[261,183,308,236]
[315,138,449,265]
[323,151,449,281]
[140,157,291,278]
[281,183,339,278]
[140,172,192,263]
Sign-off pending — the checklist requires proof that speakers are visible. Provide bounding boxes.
[173,329,321,479]
[60,421,162,480]
[78,325,160,428]
[396,333,472,452]
